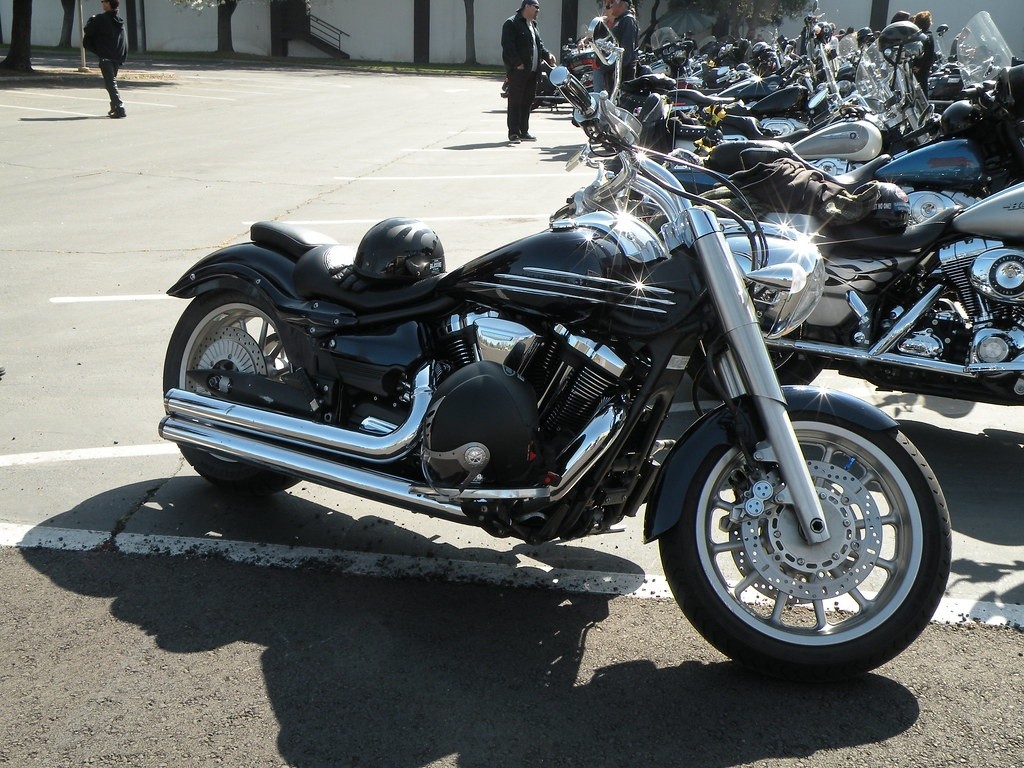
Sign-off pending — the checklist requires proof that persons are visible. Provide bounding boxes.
[500,0,557,144]
[830,23,839,55]
[579,0,640,152]
[83,0,128,119]
[950,26,975,57]
[911,10,935,100]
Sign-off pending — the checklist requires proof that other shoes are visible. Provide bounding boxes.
[108,109,126,119]
[509,135,521,144]
[520,132,536,140]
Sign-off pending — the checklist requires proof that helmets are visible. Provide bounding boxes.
[751,42,772,57]
[813,22,831,39]
[864,182,909,232]
[346,216,446,280]
[940,99,983,136]
[856,27,874,40]
[878,20,927,52]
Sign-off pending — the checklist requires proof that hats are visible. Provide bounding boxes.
[516,0,539,12]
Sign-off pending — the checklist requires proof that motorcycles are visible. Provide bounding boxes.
[158,1,1024,679]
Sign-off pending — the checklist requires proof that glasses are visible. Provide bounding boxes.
[531,5,539,9]
